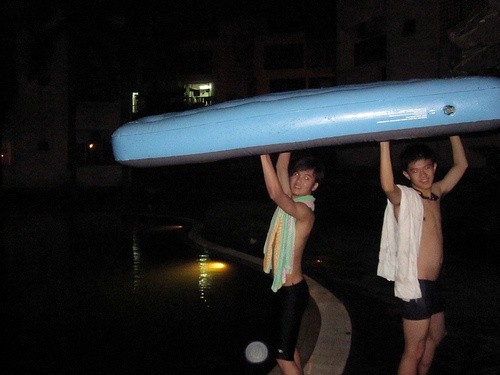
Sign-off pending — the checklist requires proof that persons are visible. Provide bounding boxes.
[260,151,320,375]
[379,135,469,374]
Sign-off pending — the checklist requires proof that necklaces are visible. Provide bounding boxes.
[412,187,440,202]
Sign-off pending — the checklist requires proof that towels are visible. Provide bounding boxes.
[376,184,425,302]
[263,194,319,294]
